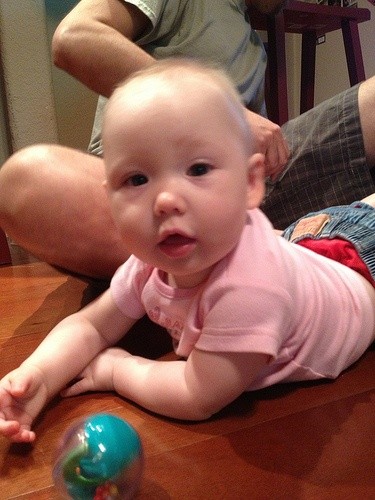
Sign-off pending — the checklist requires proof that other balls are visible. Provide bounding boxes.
[51,413,144,500]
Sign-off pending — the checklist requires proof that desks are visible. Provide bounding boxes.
[245,0,371,127]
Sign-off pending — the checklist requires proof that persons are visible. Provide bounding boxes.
[0,0,375,281]
[0,57,375,441]
